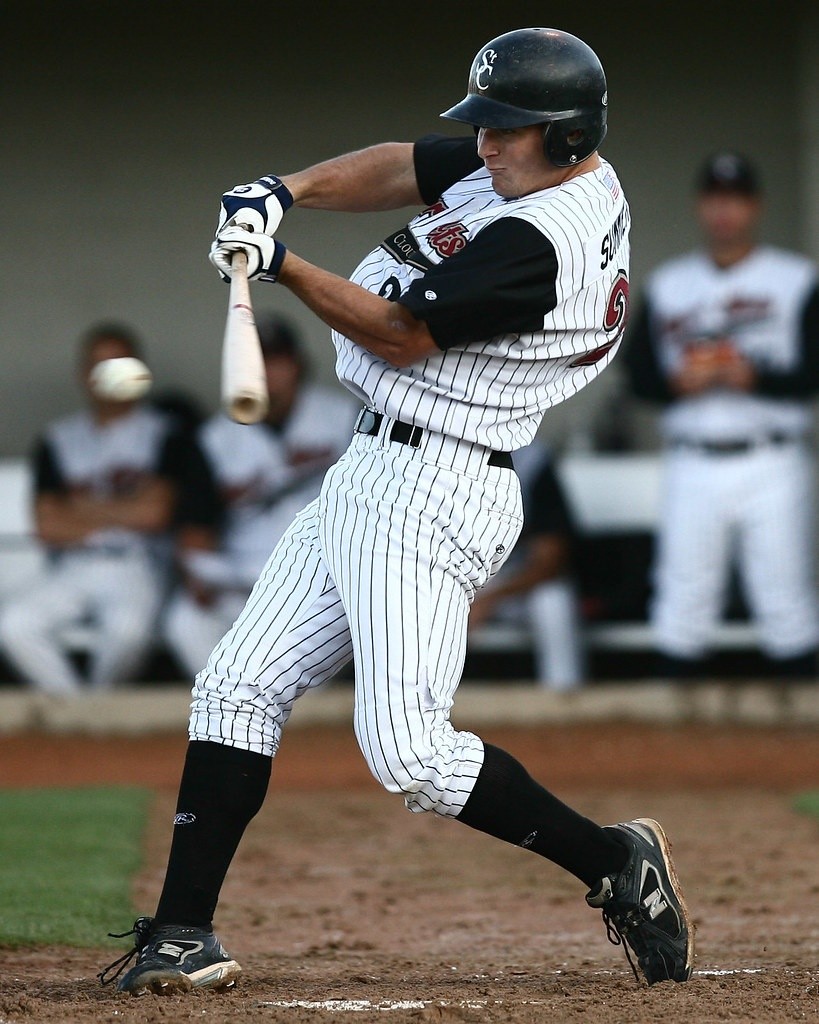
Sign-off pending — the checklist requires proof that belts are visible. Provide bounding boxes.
[678,434,786,459]
[352,403,521,472]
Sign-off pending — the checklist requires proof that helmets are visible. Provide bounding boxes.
[435,26,609,169]
[697,145,763,192]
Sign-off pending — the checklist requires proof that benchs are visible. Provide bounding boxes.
[0,464,819,657]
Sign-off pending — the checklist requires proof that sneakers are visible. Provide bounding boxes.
[585,817,698,985]
[97,915,243,1001]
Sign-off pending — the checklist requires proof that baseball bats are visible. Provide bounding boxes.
[220,252,270,426]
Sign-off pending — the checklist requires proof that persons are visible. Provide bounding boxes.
[463,435,588,687]
[158,316,356,683]
[627,144,818,686]
[97,29,689,988]
[0,324,193,696]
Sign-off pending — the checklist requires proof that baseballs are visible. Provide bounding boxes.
[88,356,153,403]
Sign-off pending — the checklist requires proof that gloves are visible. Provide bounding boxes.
[212,173,294,244]
[207,225,286,285]
